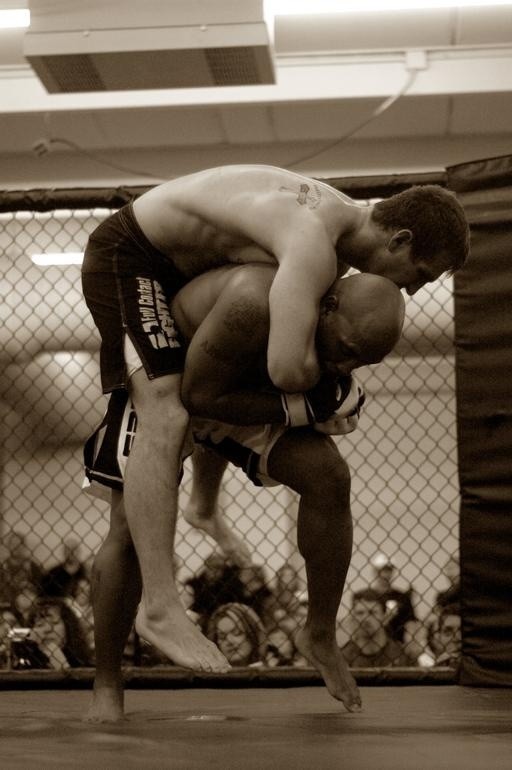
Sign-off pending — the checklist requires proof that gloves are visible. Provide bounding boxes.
[315,405,362,437]
[281,366,366,427]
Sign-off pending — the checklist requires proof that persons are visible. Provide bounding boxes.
[80,165,475,678]
[76,261,406,729]
[1,531,94,673]
[340,555,461,668]
[182,552,311,667]
